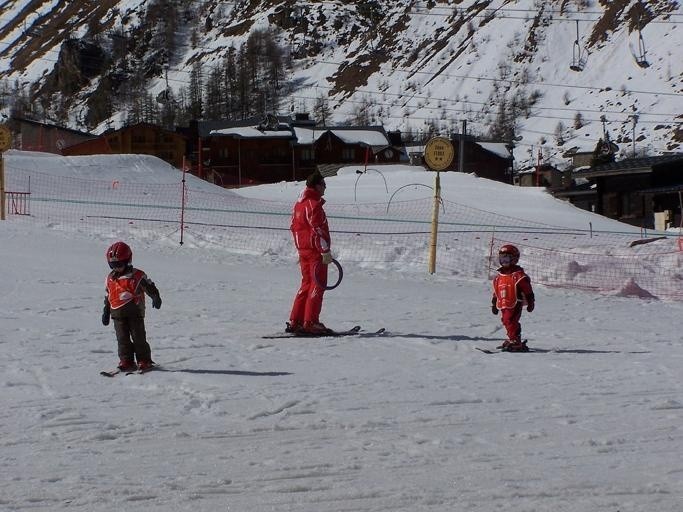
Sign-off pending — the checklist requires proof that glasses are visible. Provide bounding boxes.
[109,262,124,268]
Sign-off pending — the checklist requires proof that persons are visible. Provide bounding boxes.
[284,170,333,334]
[101,241,162,371]
[491,244,535,352]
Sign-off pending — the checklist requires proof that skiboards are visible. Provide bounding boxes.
[475,347,537,354]
[100,354,164,377]
[262,325,385,339]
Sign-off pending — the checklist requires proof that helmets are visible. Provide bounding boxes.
[106,242,132,263]
[499,245,519,267]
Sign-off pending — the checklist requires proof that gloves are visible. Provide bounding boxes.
[152,298,161,309]
[102,313,110,325]
[321,252,331,264]
[527,302,534,312]
[492,306,499,314]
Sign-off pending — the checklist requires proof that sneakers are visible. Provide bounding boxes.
[119,360,135,371]
[290,320,303,329]
[503,339,522,351]
[302,321,326,332]
[137,359,152,369]
